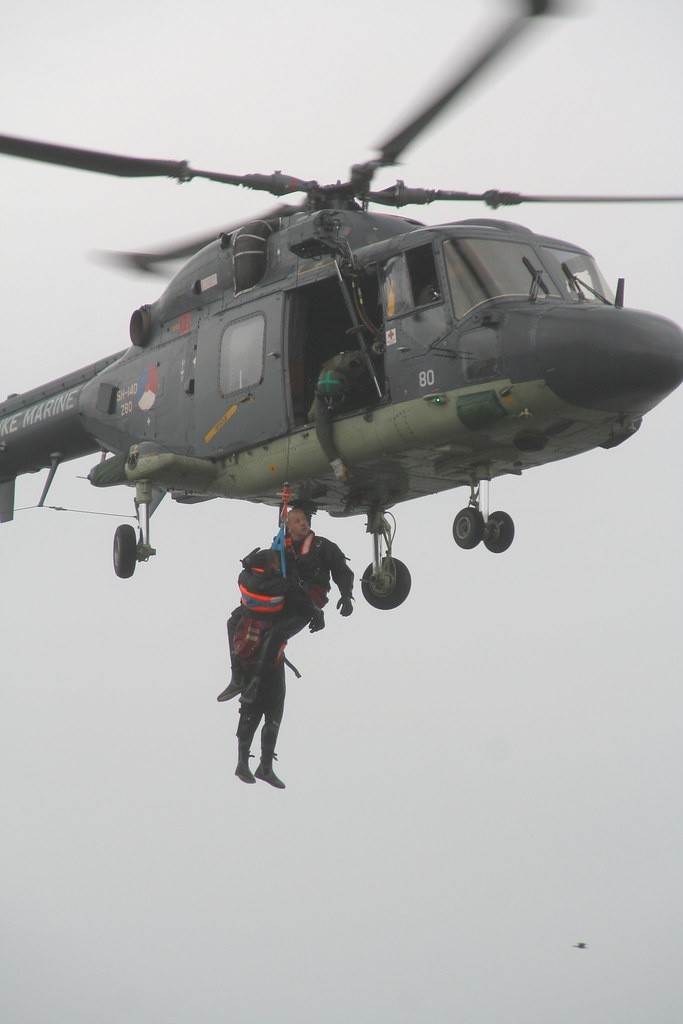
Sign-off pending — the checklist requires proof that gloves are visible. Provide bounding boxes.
[336,594,353,617]
[329,458,354,484]
[309,611,324,633]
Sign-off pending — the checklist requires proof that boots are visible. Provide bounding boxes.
[216,663,250,702]
[235,751,257,784]
[240,673,267,705]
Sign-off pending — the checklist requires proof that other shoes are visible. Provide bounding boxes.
[254,751,286,789]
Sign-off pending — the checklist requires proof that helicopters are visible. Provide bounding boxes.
[0,2,683,614]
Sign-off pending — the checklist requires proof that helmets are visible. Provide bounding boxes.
[314,370,349,395]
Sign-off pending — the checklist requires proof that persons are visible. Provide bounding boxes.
[304,350,381,485]
[235,548,326,790]
[217,507,355,705]
